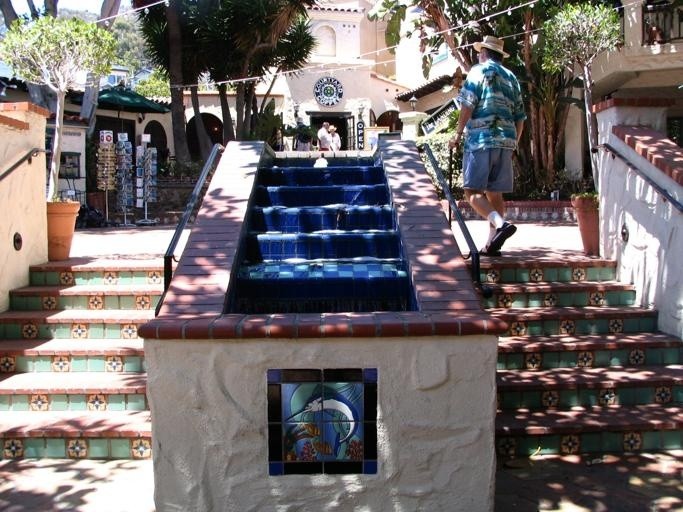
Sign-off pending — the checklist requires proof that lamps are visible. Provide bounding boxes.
[409,94,418,111]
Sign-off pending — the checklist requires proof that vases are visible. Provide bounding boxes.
[85,190,106,217]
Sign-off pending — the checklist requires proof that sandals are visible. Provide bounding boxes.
[487,221,517,252]
[475,248,503,258]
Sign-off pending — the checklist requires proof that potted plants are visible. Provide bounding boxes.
[2,13,126,261]
[521,0,622,257]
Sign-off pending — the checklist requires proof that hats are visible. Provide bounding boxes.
[472,34,511,59]
[327,124,337,132]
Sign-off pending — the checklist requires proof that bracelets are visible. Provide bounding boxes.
[457,131,462,136]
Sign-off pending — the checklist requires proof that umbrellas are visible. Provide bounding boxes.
[66,80,171,133]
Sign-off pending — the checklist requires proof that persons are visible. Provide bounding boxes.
[447,36,527,258]
[295,118,311,152]
[317,122,329,151]
[328,125,341,152]
